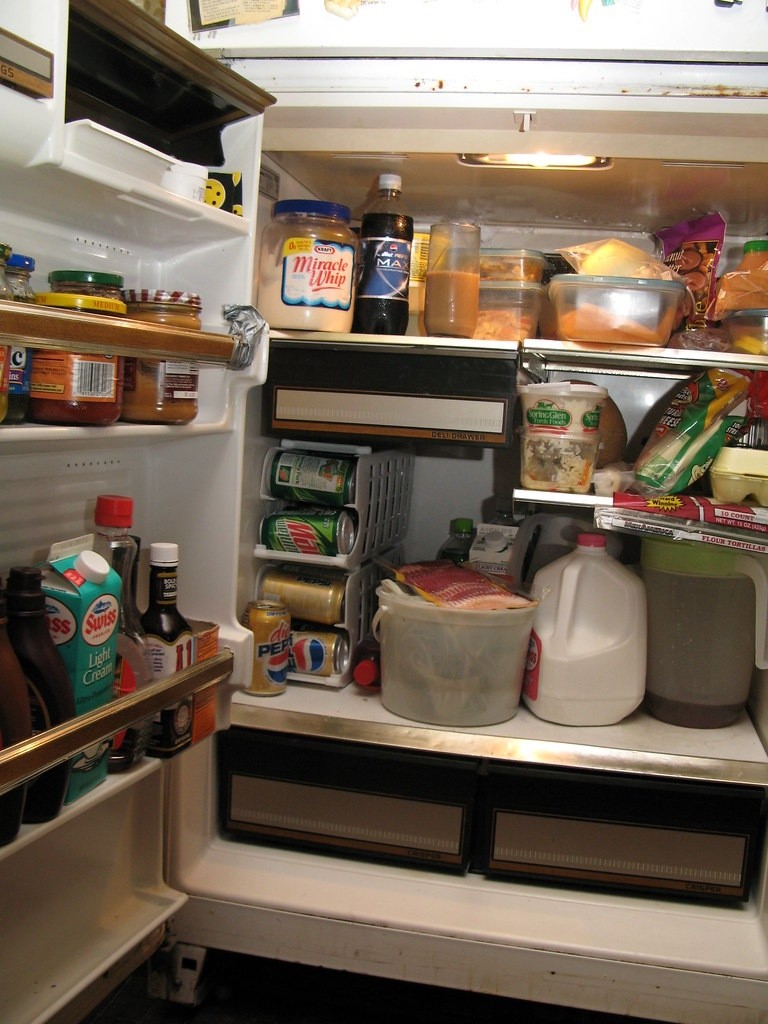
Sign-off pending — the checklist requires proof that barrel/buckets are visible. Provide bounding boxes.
[372,583,539,727]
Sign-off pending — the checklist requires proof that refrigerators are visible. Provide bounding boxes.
[0,0,768,1024]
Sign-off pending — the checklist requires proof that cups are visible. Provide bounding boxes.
[424,221,483,338]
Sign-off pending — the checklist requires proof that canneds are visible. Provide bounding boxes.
[29,293,127,425]
[260,510,355,556]
[287,630,348,677]
[0,243,14,424]
[266,451,357,506]
[3,253,34,421]
[48,270,124,304]
[242,600,290,696]
[258,564,350,624]
[119,289,201,424]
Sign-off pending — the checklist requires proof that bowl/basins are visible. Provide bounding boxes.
[515,381,610,434]
[518,431,602,493]
[724,308,768,355]
[446,245,546,282]
[464,283,543,340]
[546,272,685,349]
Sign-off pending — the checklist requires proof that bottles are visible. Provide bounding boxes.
[350,172,414,336]
[736,239,768,273]
[351,638,381,688]
[256,197,359,334]
[0,566,75,846]
[0,241,38,425]
[94,493,151,772]
[525,533,649,727]
[145,542,195,758]
[440,517,474,567]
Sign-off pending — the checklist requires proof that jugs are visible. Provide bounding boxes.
[639,530,768,730]
